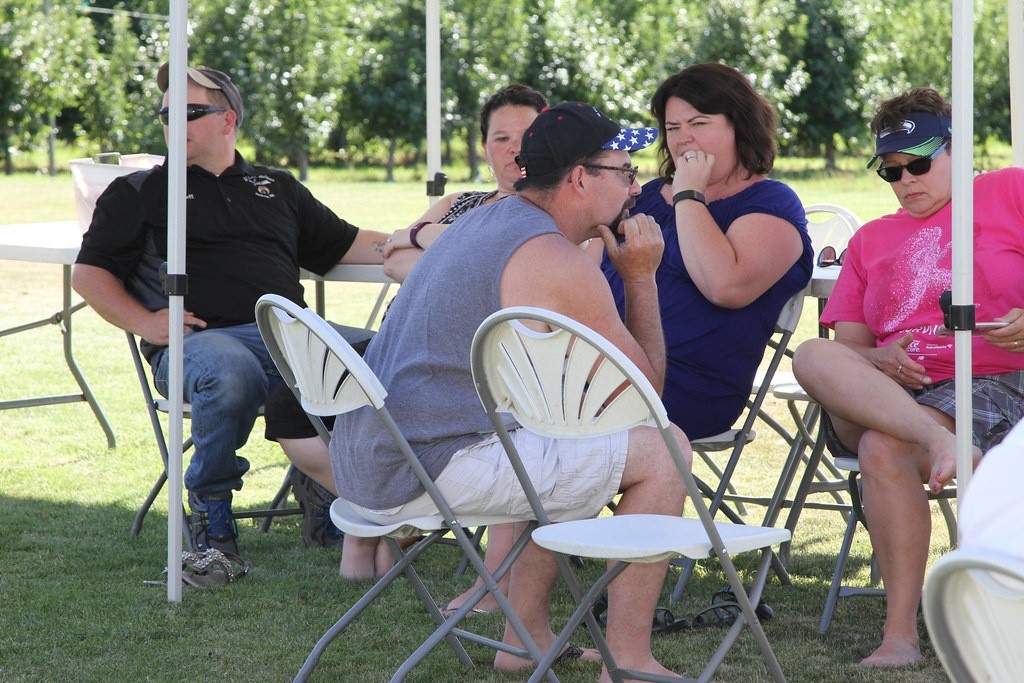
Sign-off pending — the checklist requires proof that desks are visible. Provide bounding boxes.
[0,221,858,517]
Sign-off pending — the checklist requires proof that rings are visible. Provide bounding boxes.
[898,365,903,374]
[1014,341,1020,349]
[686,154,695,159]
[386,239,393,243]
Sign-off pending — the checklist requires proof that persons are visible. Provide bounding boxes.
[330,100,694,683]
[264,64,813,623]
[71,60,392,562]
[794,85,1024,668]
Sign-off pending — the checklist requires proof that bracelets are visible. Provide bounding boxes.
[672,189,705,207]
[409,220,434,249]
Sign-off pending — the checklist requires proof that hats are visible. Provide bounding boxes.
[515,101,658,178]
[156,60,244,129]
[867,111,953,168]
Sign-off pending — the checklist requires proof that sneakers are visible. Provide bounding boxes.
[290,469,347,552]
[180,489,240,561]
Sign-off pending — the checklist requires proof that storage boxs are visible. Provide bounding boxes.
[70,153,166,234]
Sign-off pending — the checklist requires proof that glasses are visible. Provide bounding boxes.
[816,246,848,267]
[877,138,951,183]
[567,160,639,187]
[157,102,229,124]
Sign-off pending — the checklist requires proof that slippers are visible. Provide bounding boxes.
[433,602,488,619]
[490,644,584,673]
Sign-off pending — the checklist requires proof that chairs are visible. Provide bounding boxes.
[125,331,304,535]
[254,204,1023,683]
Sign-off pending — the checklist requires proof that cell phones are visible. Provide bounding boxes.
[935,322,1007,338]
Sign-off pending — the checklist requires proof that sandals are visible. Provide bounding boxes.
[144,547,249,592]
[581,595,688,637]
[690,583,772,630]
